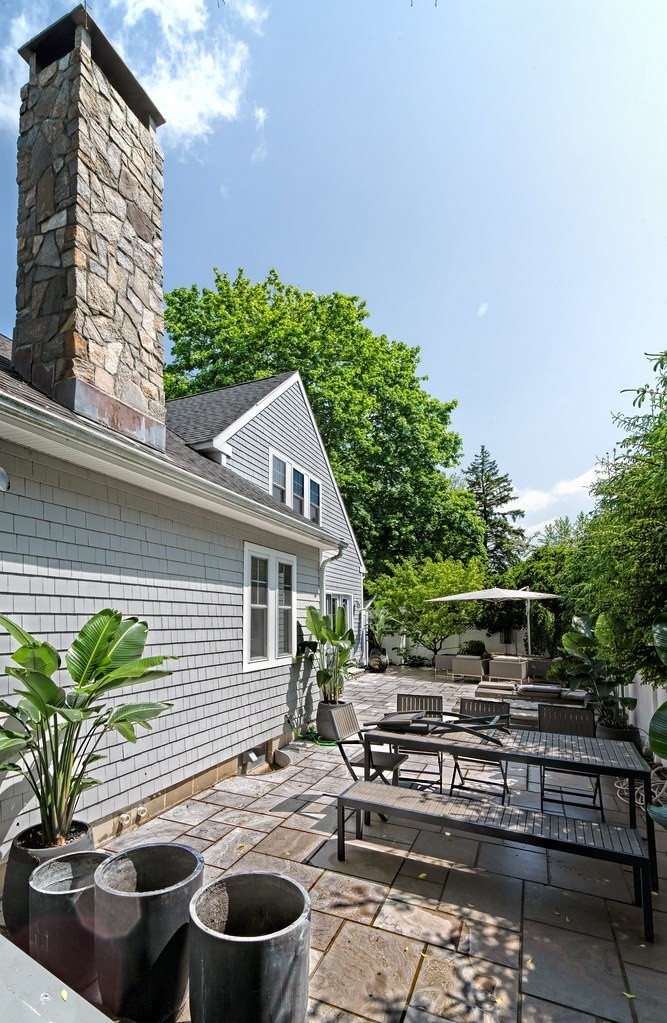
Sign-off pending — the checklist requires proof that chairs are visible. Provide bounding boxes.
[330,703,409,823]
[364,710,512,748]
[449,697,509,805]
[536,703,605,823]
[391,694,443,793]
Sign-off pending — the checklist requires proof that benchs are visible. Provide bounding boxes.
[336,779,655,945]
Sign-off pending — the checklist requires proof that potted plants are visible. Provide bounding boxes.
[297,604,356,739]
[364,600,399,673]
[0,607,180,954]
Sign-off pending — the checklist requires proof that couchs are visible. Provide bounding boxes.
[451,655,489,683]
[435,653,463,678]
[488,655,529,685]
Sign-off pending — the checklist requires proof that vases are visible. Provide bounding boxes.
[29,850,114,1010]
[187,870,312,1023]
[93,842,203,1023]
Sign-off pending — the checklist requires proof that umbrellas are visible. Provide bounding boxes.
[424,586,561,656]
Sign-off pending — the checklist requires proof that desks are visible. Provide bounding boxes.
[363,720,660,893]
